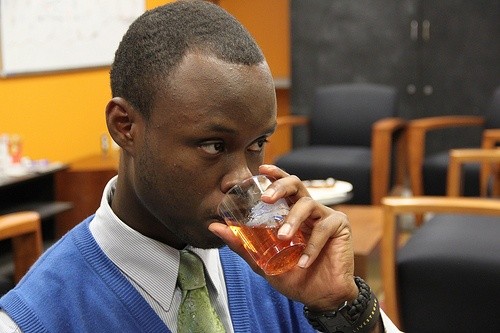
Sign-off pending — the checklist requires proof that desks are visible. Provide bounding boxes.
[330,205,385,298]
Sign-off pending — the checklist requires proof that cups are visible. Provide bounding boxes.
[217,173,306,277]
[7,136,23,164]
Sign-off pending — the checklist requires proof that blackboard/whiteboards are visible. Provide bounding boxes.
[1,2,147,78]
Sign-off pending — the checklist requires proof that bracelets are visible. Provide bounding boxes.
[302,276,370,332]
[342,293,380,333]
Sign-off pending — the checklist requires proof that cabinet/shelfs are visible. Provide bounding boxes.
[0,165,75,276]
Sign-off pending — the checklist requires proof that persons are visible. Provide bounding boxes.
[0,0,401,333]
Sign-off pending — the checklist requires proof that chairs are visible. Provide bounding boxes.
[0,211,44,284]
[266,85,500,333]
